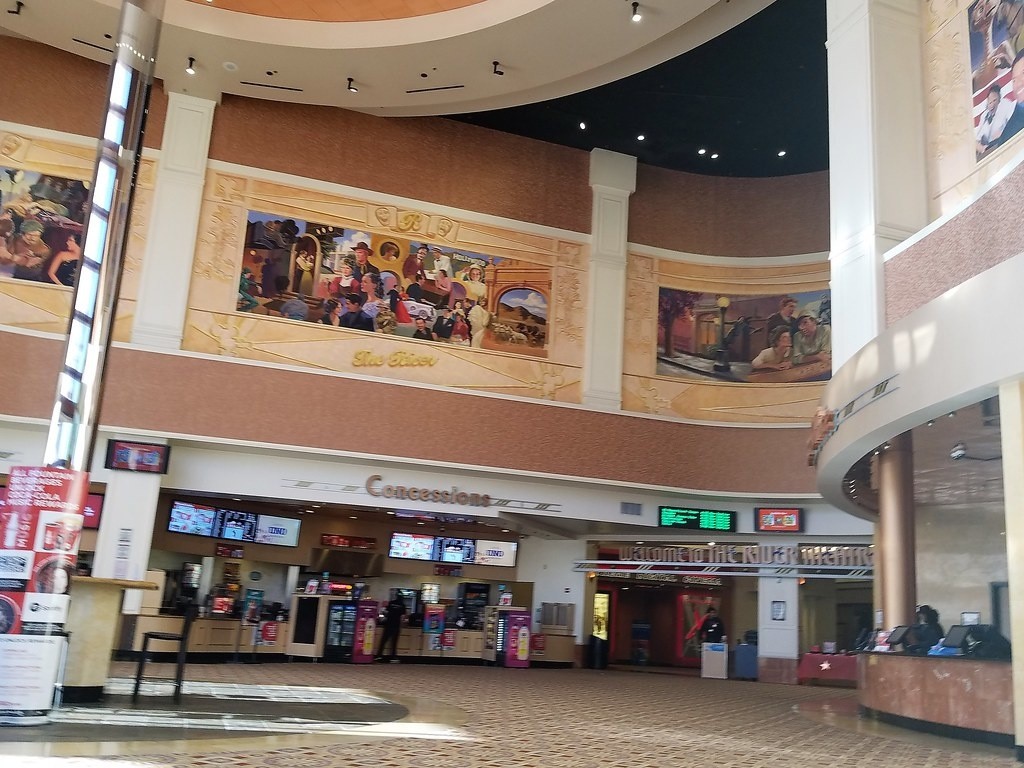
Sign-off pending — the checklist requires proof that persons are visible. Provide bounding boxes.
[374,593,406,663]
[915,605,943,653]
[699,606,728,642]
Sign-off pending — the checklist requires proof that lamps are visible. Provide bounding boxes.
[347,77,358,93]
[8,2,24,15]
[493,62,504,76]
[185,57,196,75]
[630,2,642,22]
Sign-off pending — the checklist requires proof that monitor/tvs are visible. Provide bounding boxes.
[104,438,171,474]
[918,624,1011,655]
[755,508,804,532]
[659,506,735,532]
[167,500,303,548]
[388,532,518,567]
[886,625,910,649]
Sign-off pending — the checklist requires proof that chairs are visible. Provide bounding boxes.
[131,593,198,703]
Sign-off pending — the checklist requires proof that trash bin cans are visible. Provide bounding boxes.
[590,635,608,670]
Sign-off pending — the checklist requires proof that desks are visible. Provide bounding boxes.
[796,653,858,687]
[63,572,157,709]
